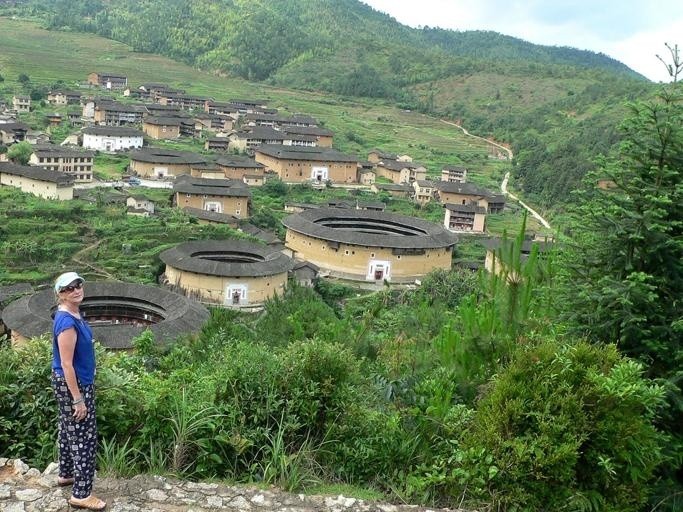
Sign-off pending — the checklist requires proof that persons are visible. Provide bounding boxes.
[49,271,107,512]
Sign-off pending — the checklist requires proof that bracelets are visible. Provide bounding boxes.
[68,397,83,406]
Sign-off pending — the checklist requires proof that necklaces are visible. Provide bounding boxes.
[63,304,80,318]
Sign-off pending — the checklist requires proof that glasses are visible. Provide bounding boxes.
[58,281,83,294]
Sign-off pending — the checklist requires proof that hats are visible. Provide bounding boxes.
[54,272,85,293]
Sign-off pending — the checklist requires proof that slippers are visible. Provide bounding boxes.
[68,496,107,512]
[57,478,76,486]
